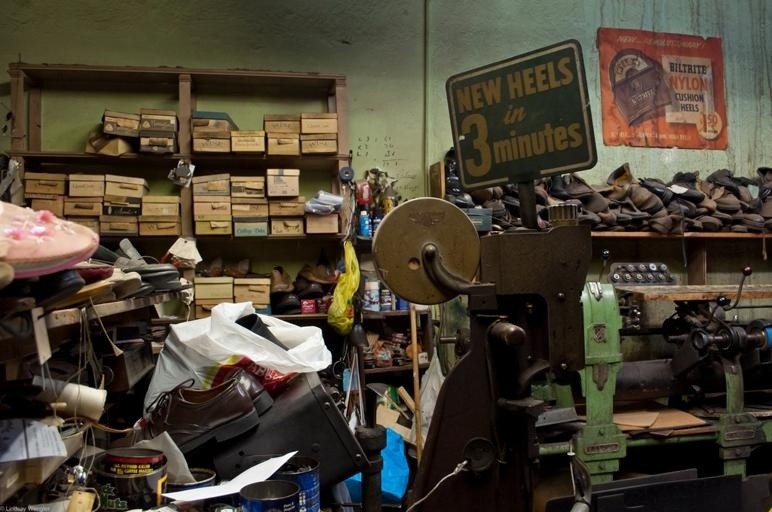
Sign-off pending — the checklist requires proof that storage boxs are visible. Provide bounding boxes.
[194,276,272,318]
[85,107,179,156]
[20,169,180,236]
[192,110,338,155]
[191,168,339,237]
[210,373,369,490]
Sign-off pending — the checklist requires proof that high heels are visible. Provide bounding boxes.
[208,256,223,277]
[232,259,250,278]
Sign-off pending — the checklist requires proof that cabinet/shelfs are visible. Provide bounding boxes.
[577,230,772,475]
[0,272,195,512]
[352,235,436,425]
[6,64,347,317]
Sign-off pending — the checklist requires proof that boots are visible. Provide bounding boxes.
[638,177,674,208]
[235,313,289,351]
[607,162,664,215]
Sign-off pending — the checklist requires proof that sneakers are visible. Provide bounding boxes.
[35,271,86,307]
[299,265,338,284]
[153,280,181,293]
[75,259,113,281]
[270,266,294,293]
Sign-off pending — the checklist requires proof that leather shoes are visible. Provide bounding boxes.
[444,146,608,231]
[247,273,271,278]
[664,167,772,232]
[223,368,274,417]
[109,378,260,456]
[91,245,179,282]
[298,283,324,297]
[133,282,153,298]
[609,196,673,234]
[275,294,300,308]
